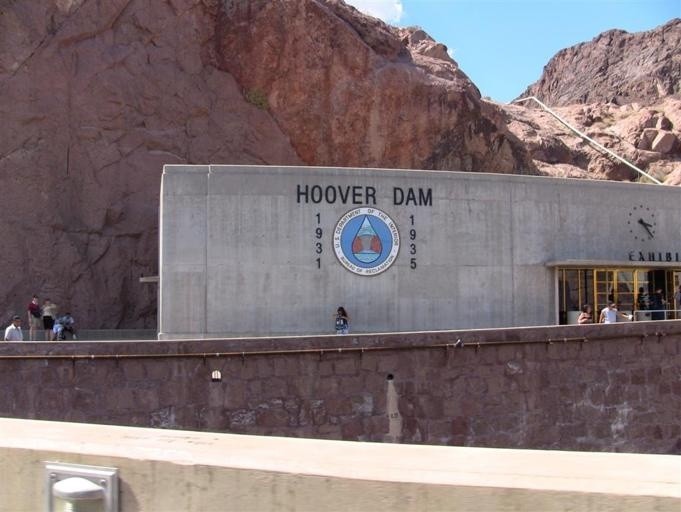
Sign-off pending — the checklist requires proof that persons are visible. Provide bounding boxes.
[598,301,631,323]
[578,304,594,324]
[4,315,25,341]
[332,307,351,335]
[40,297,57,341]
[674,284,681,319]
[63,312,78,339]
[54,314,64,342]
[637,286,648,309]
[27,294,42,340]
[651,286,667,321]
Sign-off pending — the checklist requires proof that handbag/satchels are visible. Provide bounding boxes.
[33,309,42,318]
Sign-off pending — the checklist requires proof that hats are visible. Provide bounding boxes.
[608,301,616,307]
